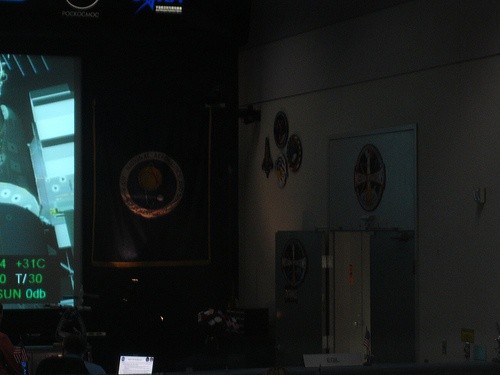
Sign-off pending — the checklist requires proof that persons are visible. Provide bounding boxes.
[34,308,109,374]
[0,302,25,374]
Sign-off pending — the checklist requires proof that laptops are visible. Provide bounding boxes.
[113,352,157,375]
